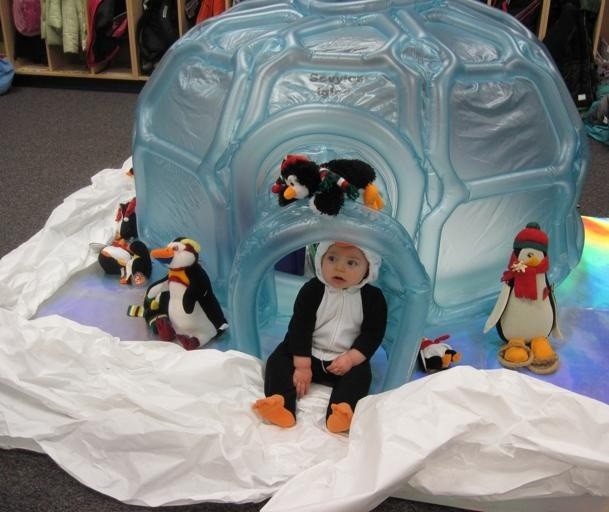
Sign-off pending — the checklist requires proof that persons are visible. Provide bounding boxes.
[252,240,388,433]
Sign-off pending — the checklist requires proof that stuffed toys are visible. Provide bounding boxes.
[150,237,231,351]
[127,274,171,335]
[89,241,152,286]
[271,154,384,217]
[417,334,462,373]
[115,197,138,246]
[483,222,564,375]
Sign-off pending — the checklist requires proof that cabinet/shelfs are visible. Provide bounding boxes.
[0,0,240,84]
[478,0,551,42]
